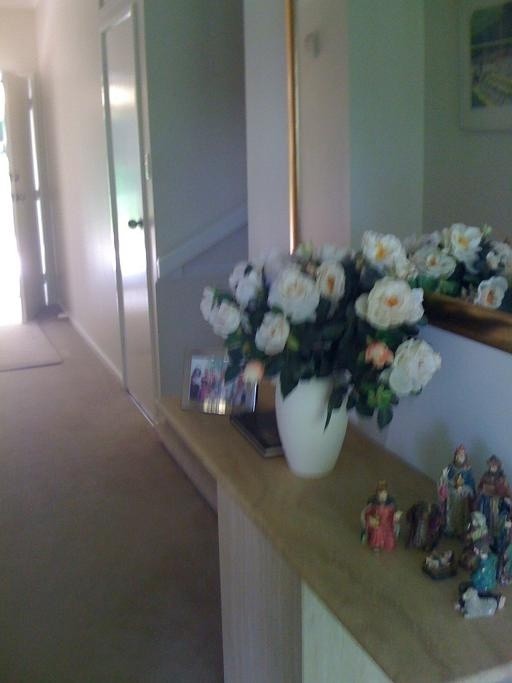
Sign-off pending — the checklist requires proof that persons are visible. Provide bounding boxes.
[358,441,512,620]
[188,363,255,407]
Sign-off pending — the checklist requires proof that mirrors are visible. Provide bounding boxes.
[285,0,512,353]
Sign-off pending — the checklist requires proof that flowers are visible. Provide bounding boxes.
[180,229,443,394]
[408,213,512,313]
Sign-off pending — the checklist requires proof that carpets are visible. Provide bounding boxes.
[1,324,65,372]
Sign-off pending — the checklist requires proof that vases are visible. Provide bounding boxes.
[270,367,355,481]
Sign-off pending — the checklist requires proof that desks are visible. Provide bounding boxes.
[154,398,510,681]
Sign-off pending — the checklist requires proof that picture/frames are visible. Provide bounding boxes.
[179,345,257,415]
[458,0,512,131]
